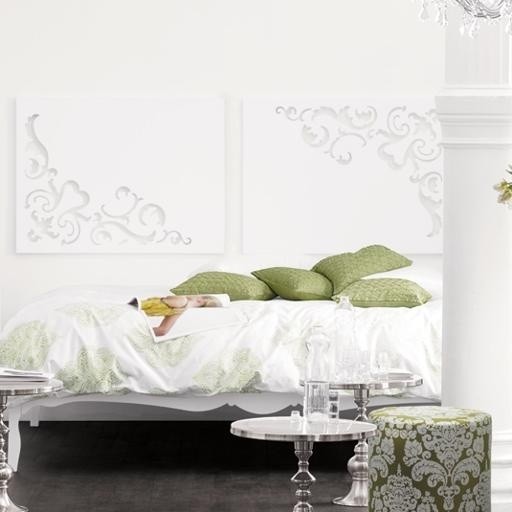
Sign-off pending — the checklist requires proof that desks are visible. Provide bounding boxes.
[1,368,64,512]
[229,416,379,512]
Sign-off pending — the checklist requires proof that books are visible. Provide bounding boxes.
[122,293,251,343]
[0,367,50,388]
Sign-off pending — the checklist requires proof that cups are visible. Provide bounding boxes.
[369,350,393,380]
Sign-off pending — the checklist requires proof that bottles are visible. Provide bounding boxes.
[289,293,362,424]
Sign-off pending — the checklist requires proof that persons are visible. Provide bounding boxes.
[140,294,222,336]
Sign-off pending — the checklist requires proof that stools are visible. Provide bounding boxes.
[365,403,492,512]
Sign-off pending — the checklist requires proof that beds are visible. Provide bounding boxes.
[0,254,444,479]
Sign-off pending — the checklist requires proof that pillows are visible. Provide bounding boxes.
[167,245,433,307]
